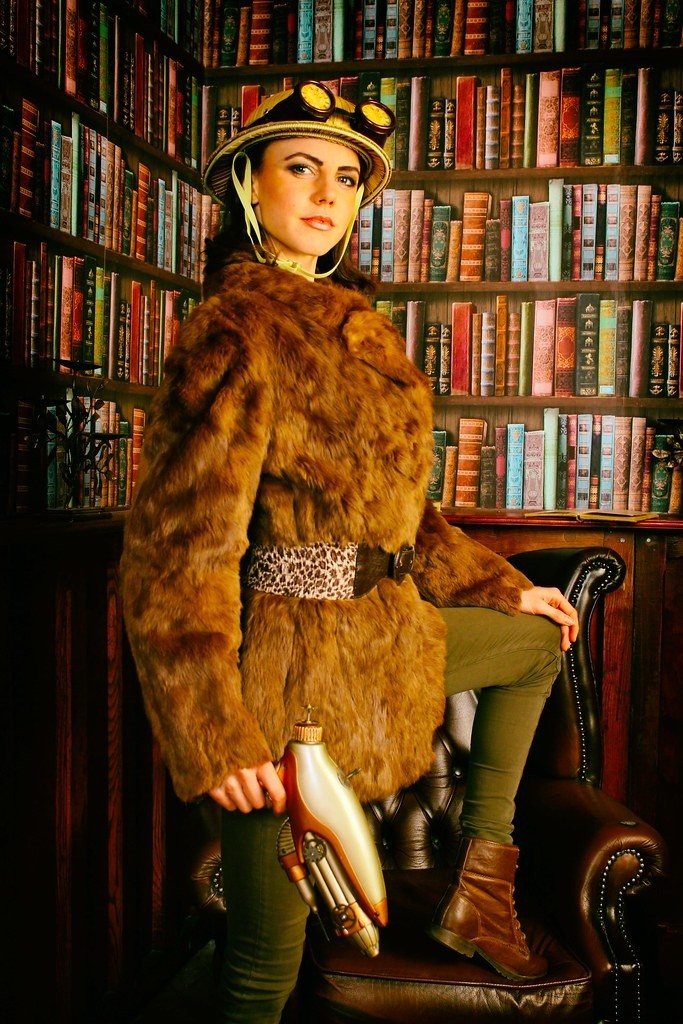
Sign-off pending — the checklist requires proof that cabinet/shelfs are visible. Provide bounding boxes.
[0,2,681,1023]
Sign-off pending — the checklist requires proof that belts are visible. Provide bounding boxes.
[252,539,414,601]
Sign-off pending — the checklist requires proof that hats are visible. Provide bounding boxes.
[202,78,396,214]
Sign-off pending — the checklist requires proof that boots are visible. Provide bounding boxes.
[426,838,551,980]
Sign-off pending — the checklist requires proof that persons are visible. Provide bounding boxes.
[119,81,578,1017]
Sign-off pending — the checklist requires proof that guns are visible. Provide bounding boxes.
[257,699,390,959]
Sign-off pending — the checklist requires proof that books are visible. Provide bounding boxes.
[0,2,681,529]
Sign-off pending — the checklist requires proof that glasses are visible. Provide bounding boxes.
[244,81,395,148]
